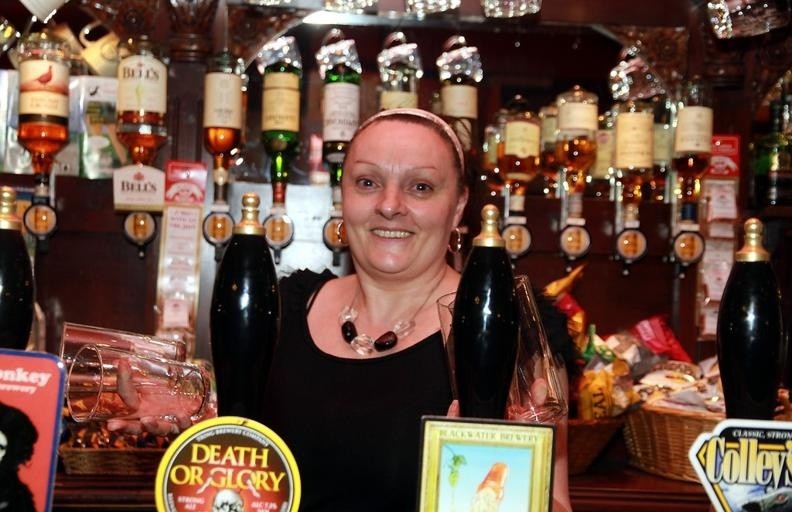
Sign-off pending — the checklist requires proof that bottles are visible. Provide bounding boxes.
[15,27,784,277]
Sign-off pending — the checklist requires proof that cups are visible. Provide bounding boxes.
[437,280,568,428]
[58,321,211,425]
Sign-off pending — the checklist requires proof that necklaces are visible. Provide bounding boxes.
[339,266,447,356]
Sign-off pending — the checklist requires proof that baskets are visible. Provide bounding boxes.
[58,441,167,477]
[567,389,792,485]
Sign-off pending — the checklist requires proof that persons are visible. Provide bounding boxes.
[107,108,572,512]
